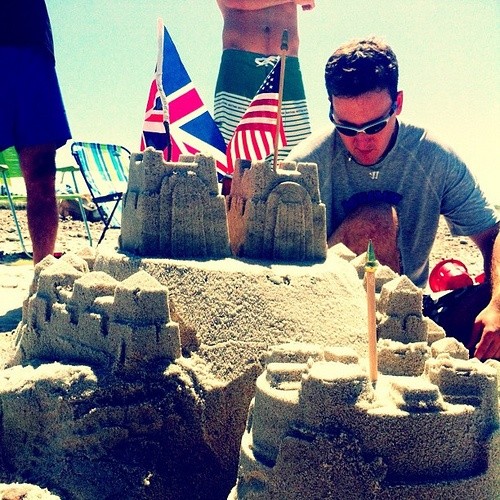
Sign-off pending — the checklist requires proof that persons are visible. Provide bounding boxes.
[214,0,315,195]
[283,40,500,362]
[0,0,72,266]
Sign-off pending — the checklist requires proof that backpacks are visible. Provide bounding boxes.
[430,282,493,358]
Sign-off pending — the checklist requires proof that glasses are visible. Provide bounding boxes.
[328,103,398,137]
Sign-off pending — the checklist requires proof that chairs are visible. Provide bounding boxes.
[0,147,92,261]
[70,142,131,244]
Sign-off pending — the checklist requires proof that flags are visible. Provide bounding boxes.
[229,53,288,178]
[140,25,227,182]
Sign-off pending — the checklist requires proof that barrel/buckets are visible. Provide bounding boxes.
[429,259,473,292]
